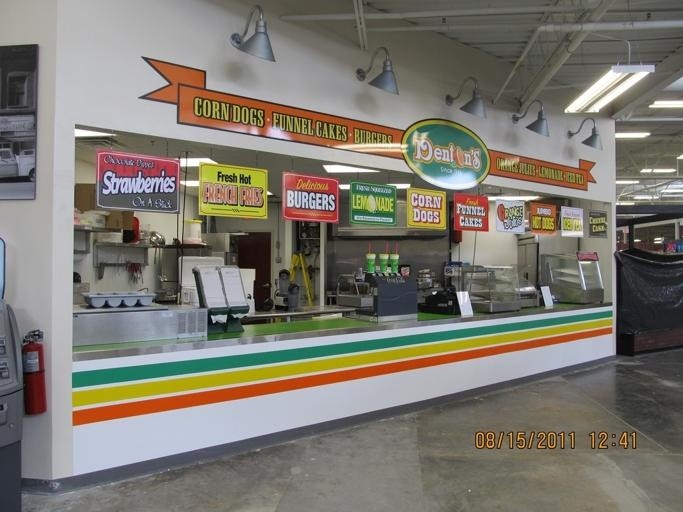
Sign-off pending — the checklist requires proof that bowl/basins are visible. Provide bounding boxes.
[151,231,166,247]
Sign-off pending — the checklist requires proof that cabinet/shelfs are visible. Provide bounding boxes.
[541,253,604,303]
[462,264,540,314]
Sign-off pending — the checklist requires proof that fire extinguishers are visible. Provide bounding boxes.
[22,330,46,415]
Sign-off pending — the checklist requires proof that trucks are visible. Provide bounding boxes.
[0,148,36,182]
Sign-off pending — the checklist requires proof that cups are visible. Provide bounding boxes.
[366,252,399,274]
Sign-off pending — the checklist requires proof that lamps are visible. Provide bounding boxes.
[230,5,276,62]
[568,117,602,152]
[355,46,399,95]
[511,98,549,136]
[447,77,486,119]
